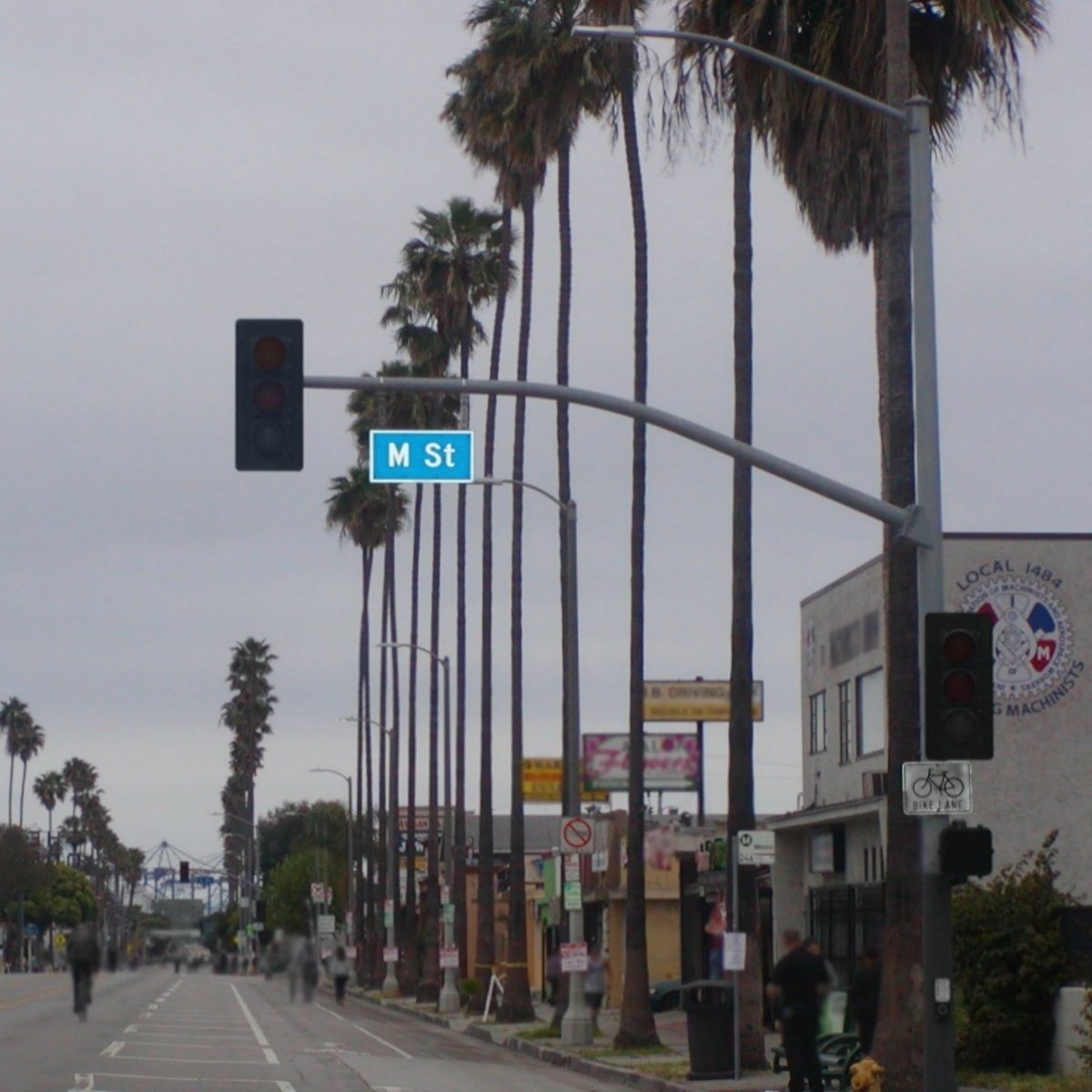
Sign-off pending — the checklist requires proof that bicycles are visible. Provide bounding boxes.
[67,958,98,1023]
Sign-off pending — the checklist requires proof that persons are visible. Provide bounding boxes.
[67,916,99,1013]
[544,948,559,1005]
[300,937,352,1006]
[582,942,612,1028]
[763,924,881,1092]
[131,940,273,980]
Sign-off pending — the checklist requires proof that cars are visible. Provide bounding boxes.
[649,978,681,1013]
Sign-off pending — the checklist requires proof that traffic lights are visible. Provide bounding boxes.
[941,826,993,879]
[234,316,304,472]
[926,609,996,760]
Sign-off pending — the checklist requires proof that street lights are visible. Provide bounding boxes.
[467,476,596,1046]
[307,766,358,984]
[210,811,262,974]
[373,641,460,1015]
[223,850,244,973]
[338,716,398,998]
[218,833,255,967]
[567,21,961,1092]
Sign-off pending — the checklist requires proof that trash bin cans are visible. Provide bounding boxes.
[683,980,738,1081]
